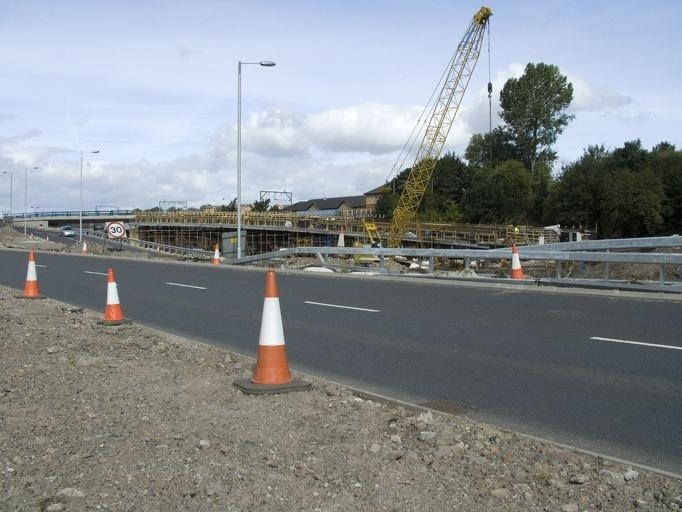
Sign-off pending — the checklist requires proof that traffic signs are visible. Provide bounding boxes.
[104,221,127,240]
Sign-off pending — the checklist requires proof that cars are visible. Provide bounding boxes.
[58,226,76,237]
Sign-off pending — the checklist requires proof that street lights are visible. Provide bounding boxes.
[79,150,99,246]
[23,166,38,236]
[3,171,12,216]
[235,59,276,256]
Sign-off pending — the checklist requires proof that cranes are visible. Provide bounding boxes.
[353,5,493,262]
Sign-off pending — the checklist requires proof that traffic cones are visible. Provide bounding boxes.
[337,226,345,246]
[252,271,292,385]
[81,242,88,254]
[104,268,126,321]
[212,243,220,264]
[23,250,41,298]
[511,243,525,279]
